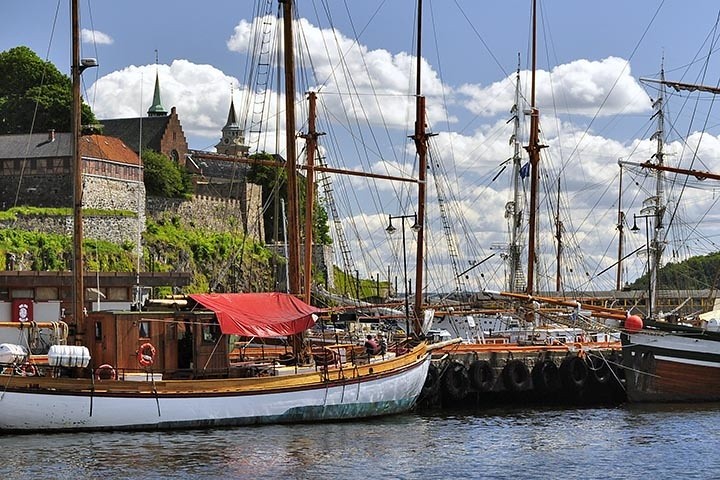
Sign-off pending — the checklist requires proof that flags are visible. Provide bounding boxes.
[518,162,530,179]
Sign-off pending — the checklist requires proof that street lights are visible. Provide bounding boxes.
[630,213,665,319]
[385,212,421,350]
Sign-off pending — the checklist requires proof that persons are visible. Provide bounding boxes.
[378,331,387,354]
[364,335,377,355]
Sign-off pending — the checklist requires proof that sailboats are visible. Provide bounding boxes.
[0,1,719,437]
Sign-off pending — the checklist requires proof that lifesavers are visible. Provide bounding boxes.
[533,359,561,393]
[422,365,440,399]
[468,360,495,391]
[139,343,156,367]
[503,359,531,394]
[613,358,626,380]
[560,354,588,390]
[589,355,613,384]
[95,364,116,380]
[440,362,471,400]
